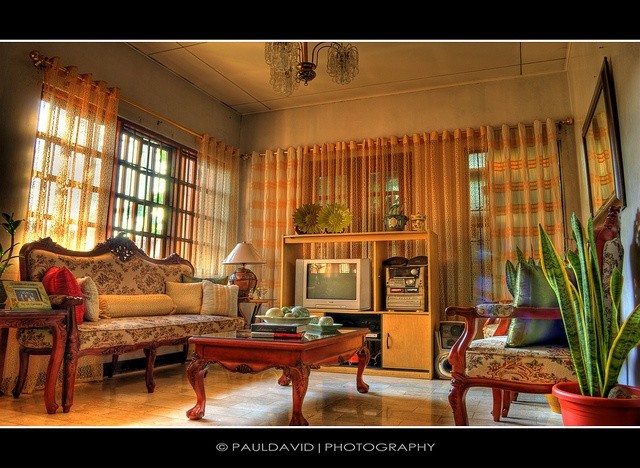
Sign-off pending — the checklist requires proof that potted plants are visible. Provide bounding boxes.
[505,245,566,413]
[539,212,640,425]
[0,211,26,309]
[384,202,407,231]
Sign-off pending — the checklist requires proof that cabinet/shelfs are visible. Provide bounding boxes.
[280,229,439,381]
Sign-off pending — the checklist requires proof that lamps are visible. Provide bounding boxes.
[266,42,360,97]
[224,241,267,303]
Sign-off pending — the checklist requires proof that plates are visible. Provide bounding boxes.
[309,322,343,335]
[255,314,313,328]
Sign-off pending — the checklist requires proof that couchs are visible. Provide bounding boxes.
[12,232,247,414]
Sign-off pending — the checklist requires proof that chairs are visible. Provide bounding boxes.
[445,205,622,426]
[483,248,578,338]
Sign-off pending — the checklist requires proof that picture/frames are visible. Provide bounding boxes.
[2,281,52,310]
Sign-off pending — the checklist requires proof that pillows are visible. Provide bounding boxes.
[42,266,85,325]
[76,276,99,323]
[99,293,177,319]
[200,280,239,318]
[166,281,203,314]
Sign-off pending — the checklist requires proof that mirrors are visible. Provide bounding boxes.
[581,57,627,227]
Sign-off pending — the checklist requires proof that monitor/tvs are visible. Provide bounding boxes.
[294,259,370,312]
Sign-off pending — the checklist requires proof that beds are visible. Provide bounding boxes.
[186,328,371,426]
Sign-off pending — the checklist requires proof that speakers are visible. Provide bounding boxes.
[434,322,465,379]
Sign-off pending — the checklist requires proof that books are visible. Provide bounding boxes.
[251,323,307,340]
